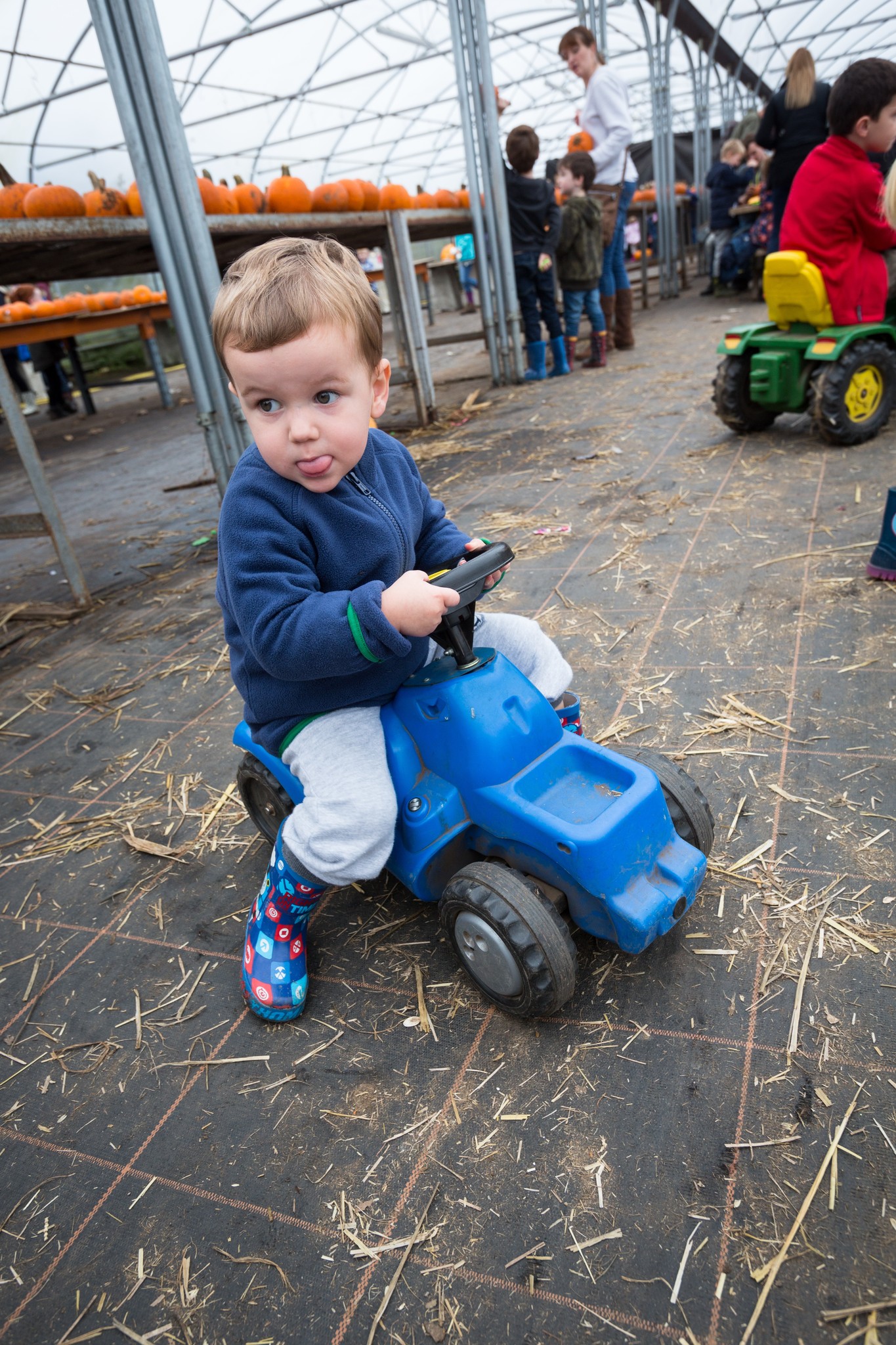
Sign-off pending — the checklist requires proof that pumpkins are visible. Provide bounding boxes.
[644,248,652,257]
[553,187,567,205]
[440,243,455,260]
[193,166,485,215]
[82,171,127,218]
[633,249,642,260]
[567,130,593,152]
[632,181,686,202]
[126,180,142,218]
[23,181,88,220]
[0,284,166,324]
[1,182,38,221]
[747,195,760,206]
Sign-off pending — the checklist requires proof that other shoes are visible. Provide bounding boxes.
[700,274,765,304]
[46,388,76,419]
[461,304,476,314]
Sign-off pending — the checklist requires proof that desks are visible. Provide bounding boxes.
[1,176,697,608]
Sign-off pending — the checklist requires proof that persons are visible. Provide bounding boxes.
[708,48,896,581]
[555,151,608,370]
[502,125,569,381]
[210,238,586,1022]
[0,284,77,419]
[558,26,637,361]
[458,233,481,316]
[355,248,378,297]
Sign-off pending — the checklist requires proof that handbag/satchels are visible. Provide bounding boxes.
[586,182,622,247]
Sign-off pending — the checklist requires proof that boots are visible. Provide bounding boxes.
[614,288,634,350]
[582,331,607,367]
[241,815,332,1024]
[548,335,570,377]
[555,690,586,740]
[577,293,616,360]
[19,390,38,415]
[866,485,896,581]
[524,342,547,380]
[563,337,578,371]
